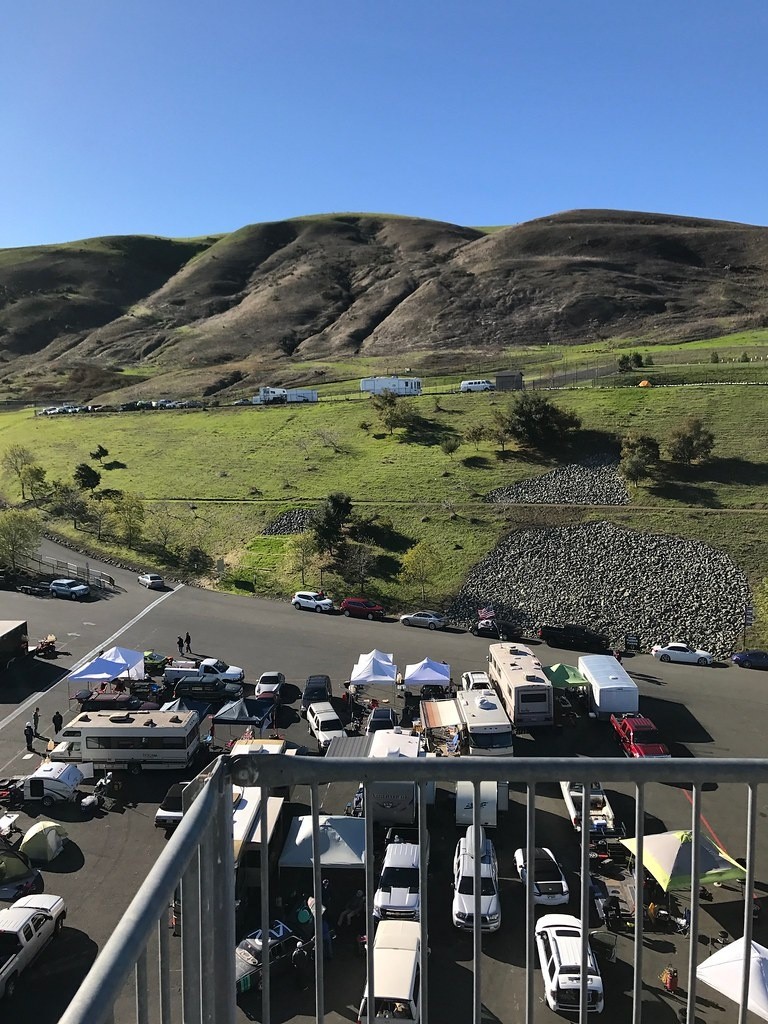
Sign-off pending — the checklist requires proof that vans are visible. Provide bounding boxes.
[460,380,495,392]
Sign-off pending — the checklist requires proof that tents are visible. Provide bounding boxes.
[277,815,366,889]
[211,697,277,747]
[0,820,71,887]
[67,657,129,711]
[619,828,747,920]
[99,645,145,670]
[542,663,593,715]
[350,649,452,705]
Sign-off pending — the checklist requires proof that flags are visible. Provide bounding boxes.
[478,605,496,619]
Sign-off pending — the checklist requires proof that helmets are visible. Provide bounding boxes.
[26,722,31,727]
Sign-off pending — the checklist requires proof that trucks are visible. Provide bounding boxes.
[360,377,422,397]
[253,390,318,404]
[485,642,555,729]
[260,387,287,403]
[456,689,515,759]
[574,654,639,716]
[50,710,202,775]
[325,726,437,829]
[171,738,299,936]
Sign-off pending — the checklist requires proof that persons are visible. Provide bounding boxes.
[53,711,63,734]
[24,721,35,752]
[177,635,184,656]
[319,589,325,598]
[184,631,192,653]
[441,660,447,664]
[32,707,42,738]
[603,895,628,930]
[614,649,623,665]
[290,878,365,992]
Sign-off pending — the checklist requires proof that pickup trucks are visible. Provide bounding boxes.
[537,624,610,652]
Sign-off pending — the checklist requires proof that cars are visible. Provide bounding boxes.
[138,573,165,589]
[652,642,714,666]
[469,619,524,641]
[0,641,674,1024]
[400,610,450,630]
[233,398,253,405]
[733,650,768,671]
[39,399,205,415]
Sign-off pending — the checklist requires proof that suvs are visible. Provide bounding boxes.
[340,597,387,621]
[291,591,333,613]
[49,578,92,600]
